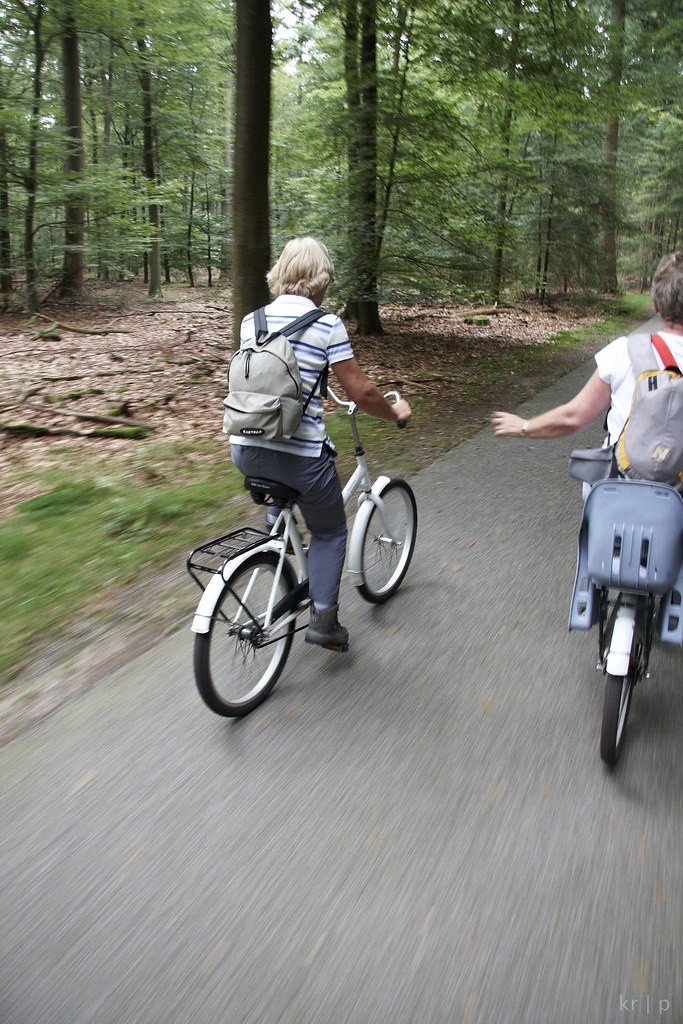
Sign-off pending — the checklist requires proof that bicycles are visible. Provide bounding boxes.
[564,420,682,762]
[184,379,420,719]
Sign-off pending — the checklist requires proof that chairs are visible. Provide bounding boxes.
[568,478,683,645]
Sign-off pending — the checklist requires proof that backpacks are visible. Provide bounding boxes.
[568,333,683,498]
[222,305,328,443]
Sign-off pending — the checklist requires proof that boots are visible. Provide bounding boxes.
[265,515,304,550]
[305,601,349,645]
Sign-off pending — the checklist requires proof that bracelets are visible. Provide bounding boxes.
[521,419,530,440]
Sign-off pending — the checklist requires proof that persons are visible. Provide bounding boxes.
[228,236,412,647]
[487,250,683,563]
